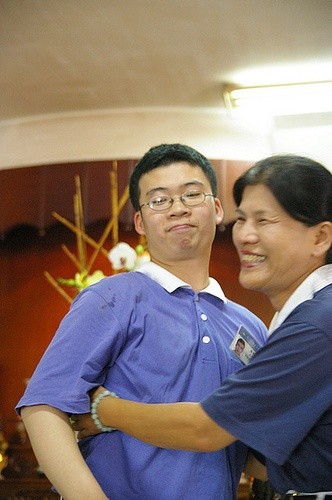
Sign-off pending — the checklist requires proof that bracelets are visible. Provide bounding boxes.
[89,390,119,433]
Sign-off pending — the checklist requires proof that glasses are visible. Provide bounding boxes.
[137,191,215,212]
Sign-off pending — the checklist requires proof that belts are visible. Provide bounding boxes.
[279,493,332,500]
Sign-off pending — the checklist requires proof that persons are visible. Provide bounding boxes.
[12,143,275,500]
[69,153,332,500]
